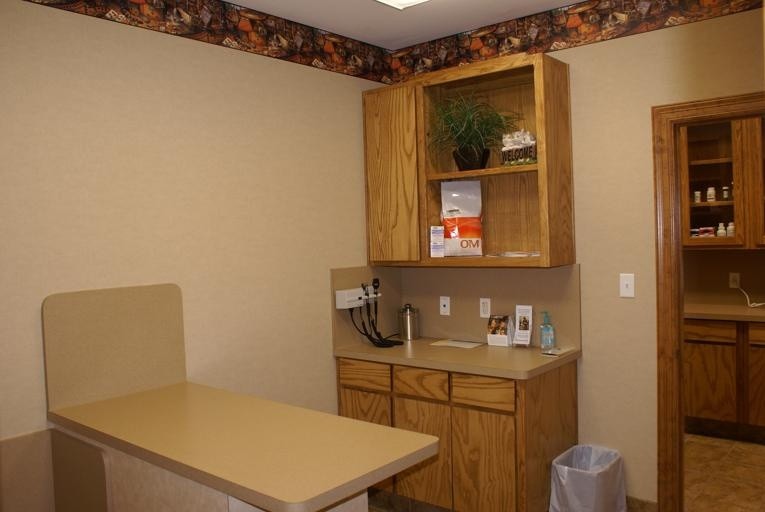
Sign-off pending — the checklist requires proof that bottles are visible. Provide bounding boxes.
[721,186,729,202]
[396,302,418,342]
[726,223,735,238]
[717,222,726,237]
[694,191,700,202]
[706,187,717,202]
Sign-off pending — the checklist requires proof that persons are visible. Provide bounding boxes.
[488,318,508,335]
[523,317,528,329]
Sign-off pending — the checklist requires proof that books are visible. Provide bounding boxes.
[499,249,541,257]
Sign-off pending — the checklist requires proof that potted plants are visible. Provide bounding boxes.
[427,90,525,171]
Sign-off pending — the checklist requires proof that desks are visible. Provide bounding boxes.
[46,383,441,510]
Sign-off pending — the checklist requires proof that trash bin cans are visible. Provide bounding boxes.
[549,444,621,512]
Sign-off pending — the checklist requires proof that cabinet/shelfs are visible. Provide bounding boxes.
[362,53,576,269]
[681,316,765,445]
[334,356,578,512]
[679,110,765,253]
